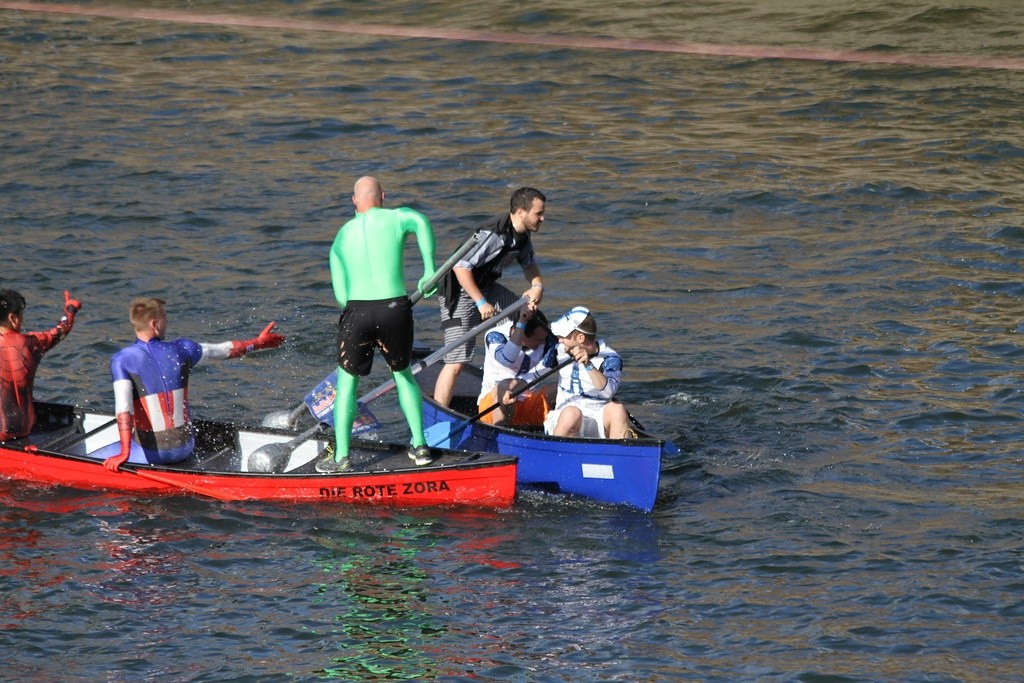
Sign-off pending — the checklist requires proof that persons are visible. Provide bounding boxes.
[315,176,439,472]
[85,295,285,472]
[503,306,631,438]
[0,287,82,442]
[476,308,559,427]
[435,186,546,407]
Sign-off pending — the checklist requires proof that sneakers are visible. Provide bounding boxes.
[315,445,354,472]
[408,443,433,465]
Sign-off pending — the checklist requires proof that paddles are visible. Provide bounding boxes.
[427,346,588,443]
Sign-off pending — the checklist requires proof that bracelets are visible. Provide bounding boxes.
[475,298,487,307]
[516,322,525,329]
[532,284,544,290]
[585,361,594,371]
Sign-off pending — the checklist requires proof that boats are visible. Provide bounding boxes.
[0,399,523,510]
[410,339,665,514]
